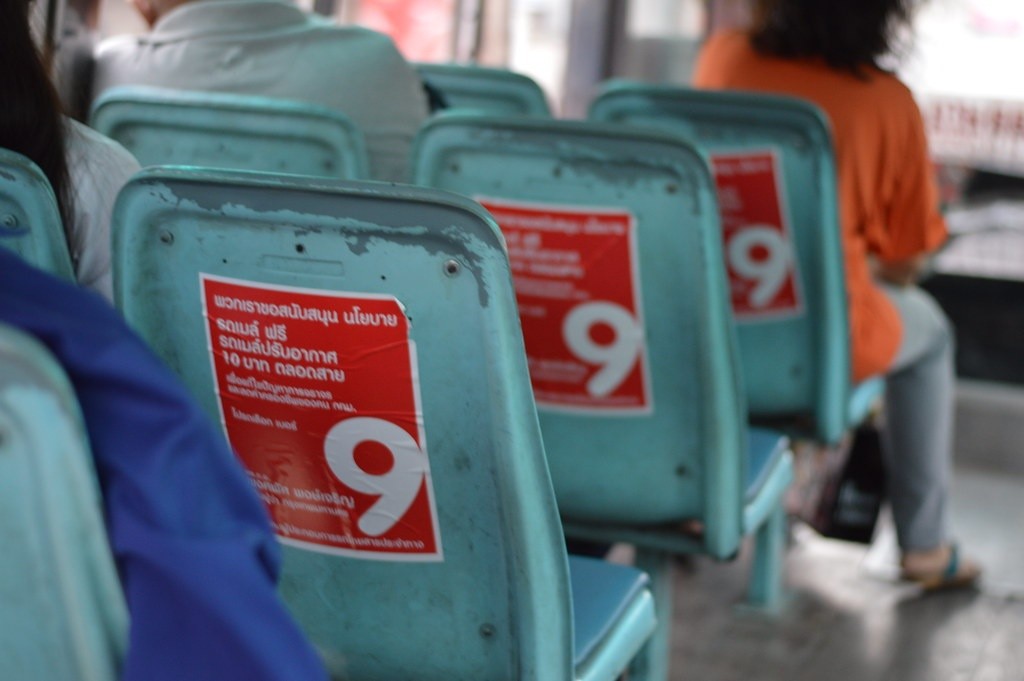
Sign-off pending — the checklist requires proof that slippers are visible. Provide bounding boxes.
[897,542,983,594]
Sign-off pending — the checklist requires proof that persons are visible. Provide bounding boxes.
[90,1,428,188]
[0,3,146,311]
[692,1,982,590]
[6,249,326,681]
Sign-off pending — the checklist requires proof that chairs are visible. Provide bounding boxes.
[0,61,888,681]
